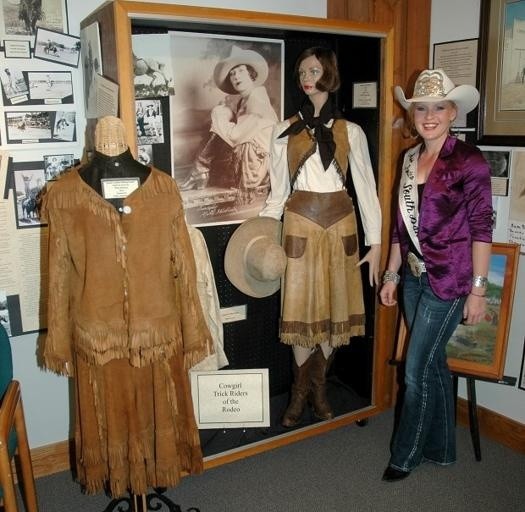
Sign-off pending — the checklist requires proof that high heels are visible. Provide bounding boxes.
[175,166,210,191]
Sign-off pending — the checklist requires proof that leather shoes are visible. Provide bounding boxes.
[378,464,412,483]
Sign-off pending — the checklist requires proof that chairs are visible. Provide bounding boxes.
[0,322,42,512]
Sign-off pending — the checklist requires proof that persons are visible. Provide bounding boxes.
[37,113,216,496]
[4,67,20,95]
[137,108,146,136]
[46,39,50,55]
[178,44,275,191]
[378,70,493,482]
[145,106,161,139]
[256,46,380,425]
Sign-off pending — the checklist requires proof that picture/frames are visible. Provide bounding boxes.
[392,239,522,382]
[465,1,525,147]
[431,36,479,87]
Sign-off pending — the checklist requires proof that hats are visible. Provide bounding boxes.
[211,43,271,97]
[393,67,481,118]
[220,214,287,299]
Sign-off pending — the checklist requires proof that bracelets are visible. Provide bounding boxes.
[383,270,401,283]
[471,291,487,297]
[472,275,489,288]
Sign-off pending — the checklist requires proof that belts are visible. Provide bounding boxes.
[405,253,427,278]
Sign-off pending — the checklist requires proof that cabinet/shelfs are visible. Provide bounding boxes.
[75,1,397,485]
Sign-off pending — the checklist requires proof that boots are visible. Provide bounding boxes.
[280,345,317,429]
[306,342,338,422]
